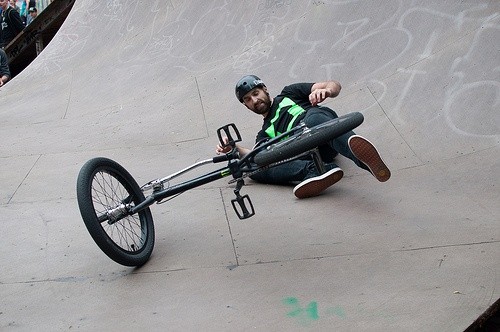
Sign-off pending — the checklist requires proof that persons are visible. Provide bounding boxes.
[216,74,391,199]
[9,0,35,25]
[0,0,25,50]
[28,7,38,24]
[0,46,10,87]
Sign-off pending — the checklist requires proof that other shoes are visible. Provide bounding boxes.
[292,162,344,198]
[348,135,391,182]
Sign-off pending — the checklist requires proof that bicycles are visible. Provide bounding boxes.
[75,111,365,267]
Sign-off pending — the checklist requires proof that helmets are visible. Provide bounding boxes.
[235,75,267,105]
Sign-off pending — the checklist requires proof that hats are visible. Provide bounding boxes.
[28,7,37,12]
